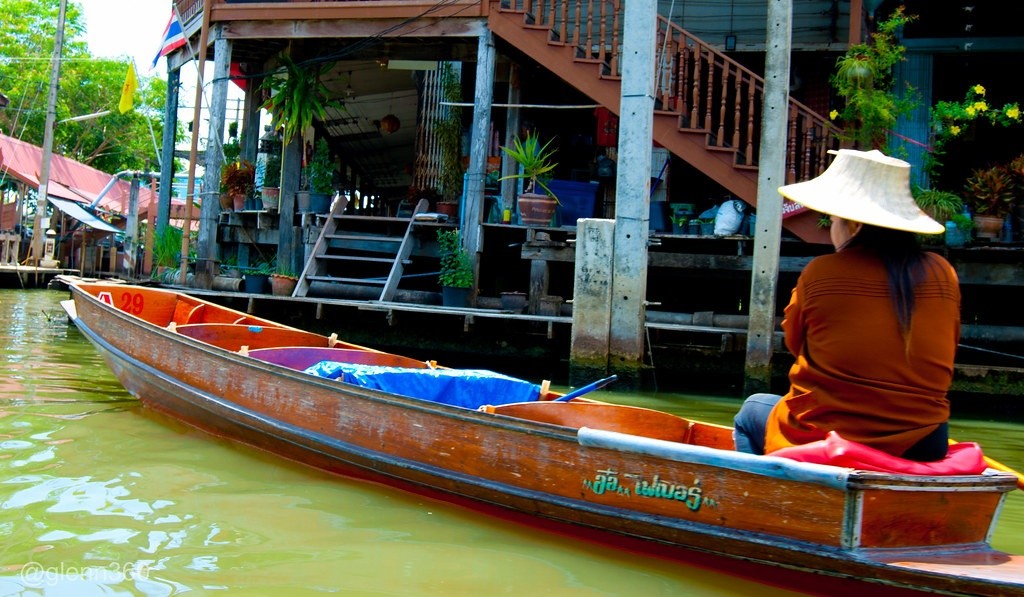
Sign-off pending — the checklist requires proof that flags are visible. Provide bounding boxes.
[118,59,142,115]
[152,8,190,69]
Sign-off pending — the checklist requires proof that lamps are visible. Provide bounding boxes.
[725,0,737,51]
[380,83,401,134]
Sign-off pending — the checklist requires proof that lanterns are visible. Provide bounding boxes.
[382,114,401,134]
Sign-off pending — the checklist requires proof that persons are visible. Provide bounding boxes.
[733,149,961,462]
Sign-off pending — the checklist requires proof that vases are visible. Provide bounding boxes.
[500,291,564,318]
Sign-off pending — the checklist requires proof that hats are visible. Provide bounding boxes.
[775,147,948,236]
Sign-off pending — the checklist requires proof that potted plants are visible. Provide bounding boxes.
[669,214,714,236]
[145,225,183,285]
[219,121,341,215]
[437,228,475,308]
[497,126,565,225]
[241,260,300,297]
[432,116,460,224]
[908,155,1024,250]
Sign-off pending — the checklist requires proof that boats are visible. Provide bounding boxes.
[58,282,1024,597]
